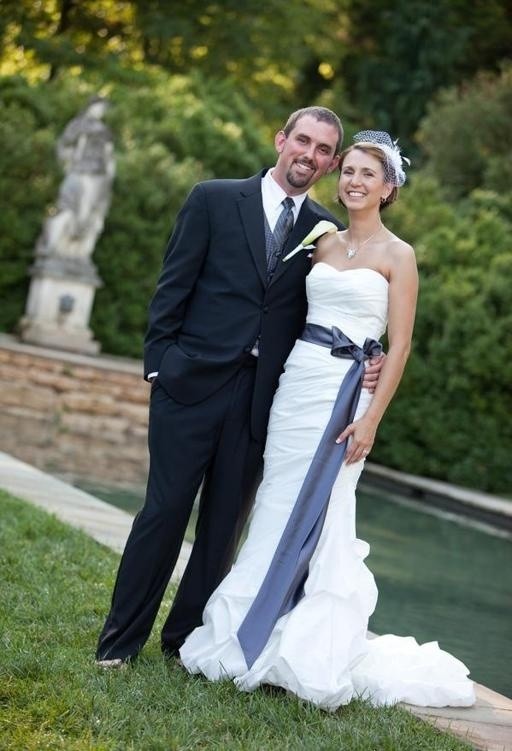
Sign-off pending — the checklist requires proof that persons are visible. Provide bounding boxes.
[91,105,344,683]
[189,129,474,714]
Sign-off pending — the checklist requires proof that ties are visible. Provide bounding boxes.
[273,195,295,251]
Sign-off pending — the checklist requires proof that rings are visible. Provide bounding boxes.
[362,450,367,454]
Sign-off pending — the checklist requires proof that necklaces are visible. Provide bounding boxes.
[342,221,384,259]
